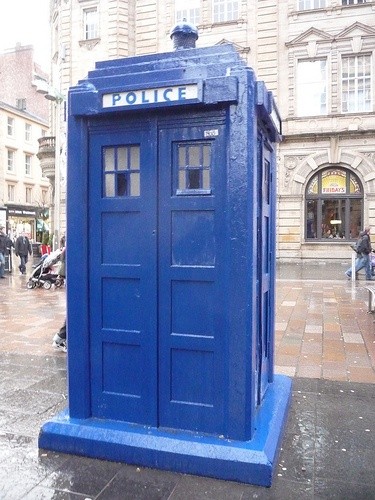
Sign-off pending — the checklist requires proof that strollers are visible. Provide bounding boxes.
[24,253,65,291]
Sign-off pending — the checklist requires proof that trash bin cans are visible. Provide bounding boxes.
[32,244,41,257]
[40,245,50,257]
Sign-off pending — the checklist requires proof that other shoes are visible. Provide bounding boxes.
[22,272,27,275]
[345,272,352,279]
[0,276,7,279]
[366,276,375,280]
[18,266,22,271]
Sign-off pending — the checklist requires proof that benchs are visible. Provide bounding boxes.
[365,285,375,324]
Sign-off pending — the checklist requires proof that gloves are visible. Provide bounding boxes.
[16,253,19,257]
[30,252,32,255]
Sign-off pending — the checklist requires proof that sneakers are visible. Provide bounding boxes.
[53,340,66,353]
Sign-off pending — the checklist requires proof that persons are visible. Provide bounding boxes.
[0,225,11,278]
[323,228,352,239]
[15,231,32,274]
[345,227,375,280]
[51,236,67,352]
[39,243,50,256]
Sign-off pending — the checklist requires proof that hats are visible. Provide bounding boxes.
[0,226,5,230]
